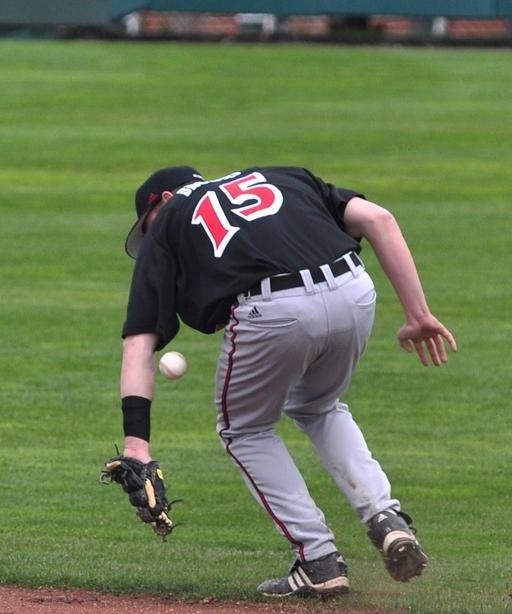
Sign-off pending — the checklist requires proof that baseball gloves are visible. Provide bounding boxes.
[104,456,171,536]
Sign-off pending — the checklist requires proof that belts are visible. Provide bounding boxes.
[245,255,360,297]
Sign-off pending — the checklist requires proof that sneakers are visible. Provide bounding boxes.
[369,511,429,583]
[258,555,352,601]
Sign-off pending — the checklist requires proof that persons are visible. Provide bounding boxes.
[101,165,460,595]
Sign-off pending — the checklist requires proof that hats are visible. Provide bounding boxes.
[125,166,204,262]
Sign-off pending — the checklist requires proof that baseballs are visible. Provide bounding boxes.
[159,352,187,379]
